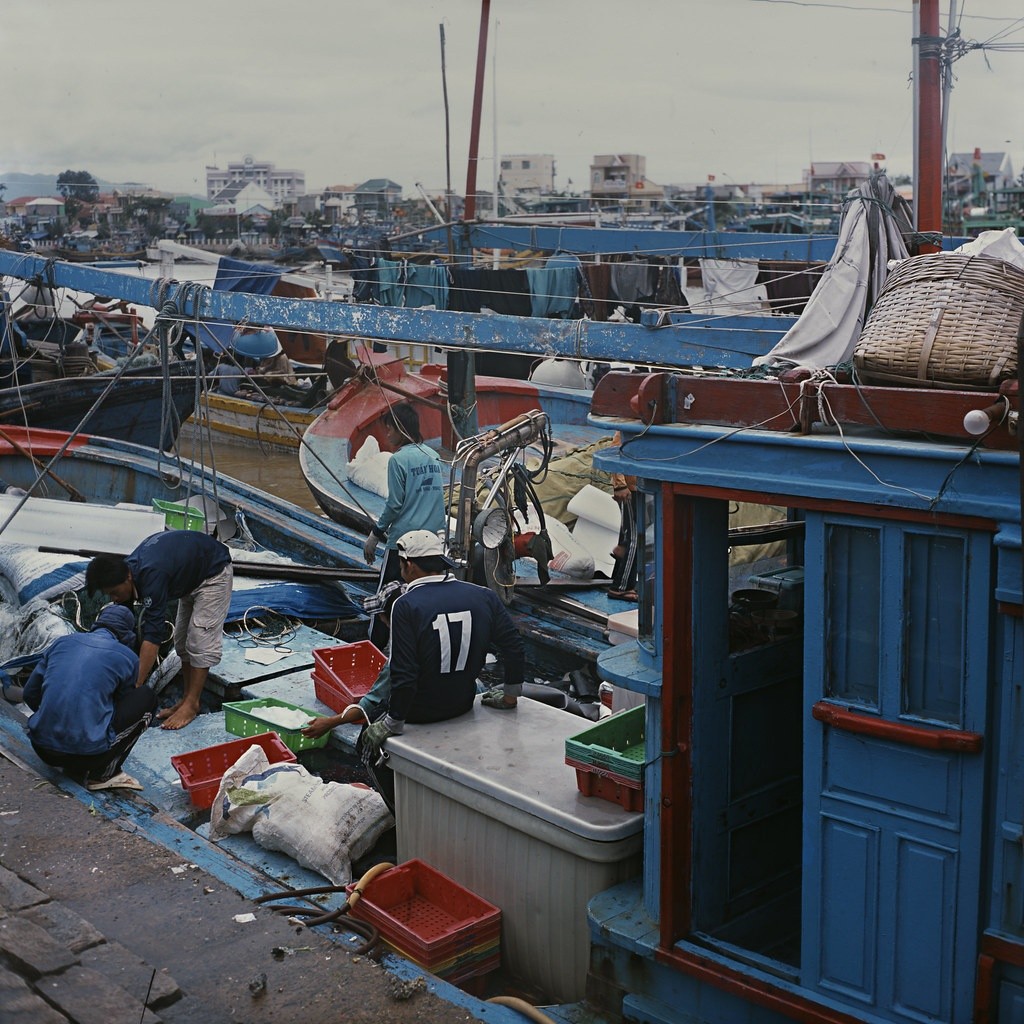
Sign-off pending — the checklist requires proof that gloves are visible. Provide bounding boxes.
[361,721,397,757]
[481,687,518,710]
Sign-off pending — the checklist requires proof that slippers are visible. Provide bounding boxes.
[87,771,144,790]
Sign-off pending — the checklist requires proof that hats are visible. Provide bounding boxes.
[92,605,137,647]
[396,529,458,570]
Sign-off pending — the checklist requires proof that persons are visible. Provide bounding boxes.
[85,529,234,730]
[608,431,641,602]
[209,358,242,397]
[23,605,158,791]
[262,350,298,386]
[301,405,527,853]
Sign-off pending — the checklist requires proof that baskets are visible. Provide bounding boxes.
[222,697,331,752]
[170,730,297,810]
[311,670,367,724]
[564,705,645,813]
[346,858,502,996]
[312,640,389,703]
[853,253,1023,391]
[151,497,205,532]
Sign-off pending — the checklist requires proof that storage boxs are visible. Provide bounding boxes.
[222,697,331,752]
[171,731,298,810]
[565,703,654,812]
[151,498,205,532]
[309,640,389,724]
[748,566,804,611]
[346,858,502,999]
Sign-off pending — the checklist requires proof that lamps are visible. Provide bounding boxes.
[230,324,282,359]
[20,284,57,307]
[530,357,587,393]
[543,250,583,269]
[471,505,510,551]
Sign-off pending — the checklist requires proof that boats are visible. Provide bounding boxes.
[1,0,1024,1023]
